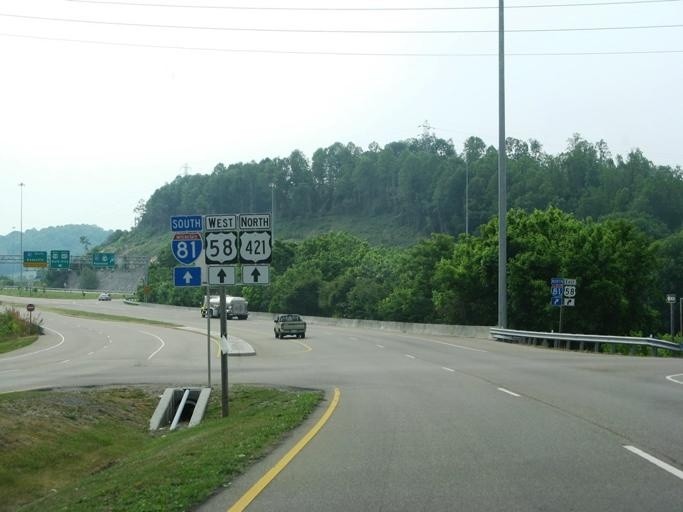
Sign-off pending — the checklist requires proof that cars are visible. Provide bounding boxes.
[98,293,111,302]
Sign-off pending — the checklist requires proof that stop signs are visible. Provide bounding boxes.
[26,303,35,312]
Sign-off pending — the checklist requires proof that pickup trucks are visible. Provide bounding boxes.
[271,314,307,340]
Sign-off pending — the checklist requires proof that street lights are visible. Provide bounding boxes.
[267,181,277,244]
[19,181,25,281]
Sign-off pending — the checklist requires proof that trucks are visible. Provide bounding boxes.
[197,294,250,321]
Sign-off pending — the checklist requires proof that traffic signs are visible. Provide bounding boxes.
[23,250,47,268]
[50,249,70,270]
[90,252,115,267]
